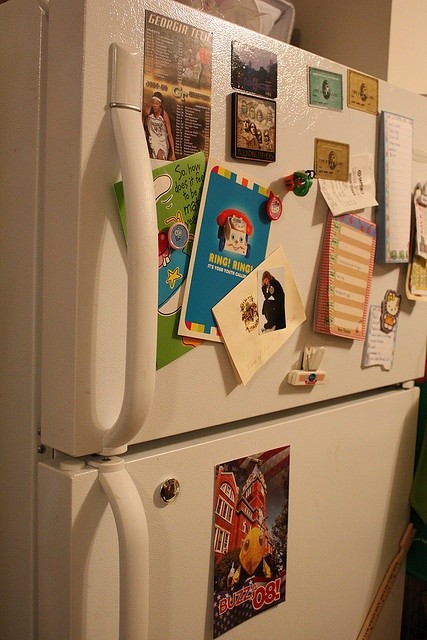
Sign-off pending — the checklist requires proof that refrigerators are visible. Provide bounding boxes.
[0,0,427,640]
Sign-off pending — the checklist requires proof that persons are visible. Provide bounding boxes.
[259,272,286,331]
[235,99,274,152]
[146,92,176,160]
